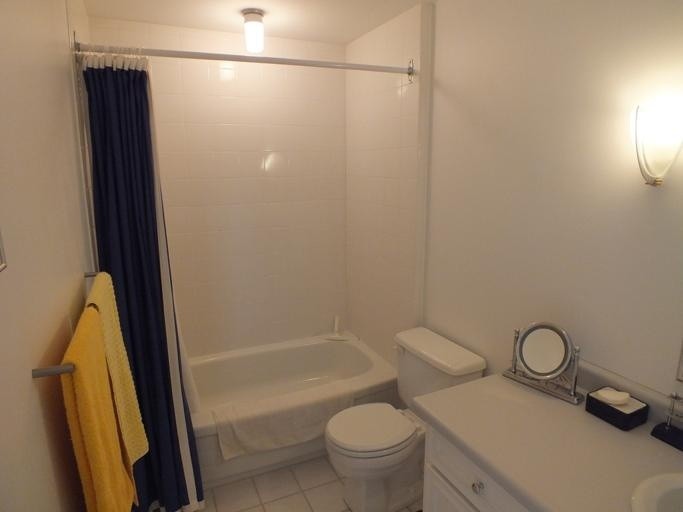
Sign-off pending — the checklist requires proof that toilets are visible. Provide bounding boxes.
[324,327,487,512]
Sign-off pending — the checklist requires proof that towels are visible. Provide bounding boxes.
[212,377,354,459]
[83,269,151,467]
[60,305,135,512]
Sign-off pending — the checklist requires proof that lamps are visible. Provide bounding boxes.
[633,90,683,186]
[241,7,266,53]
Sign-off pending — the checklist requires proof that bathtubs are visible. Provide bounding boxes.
[185,331,397,437]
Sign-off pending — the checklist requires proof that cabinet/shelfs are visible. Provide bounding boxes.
[421,423,527,511]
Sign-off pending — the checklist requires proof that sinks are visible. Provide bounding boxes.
[630,473,683,512]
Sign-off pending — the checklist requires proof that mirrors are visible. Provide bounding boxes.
[501,318,585,406]
